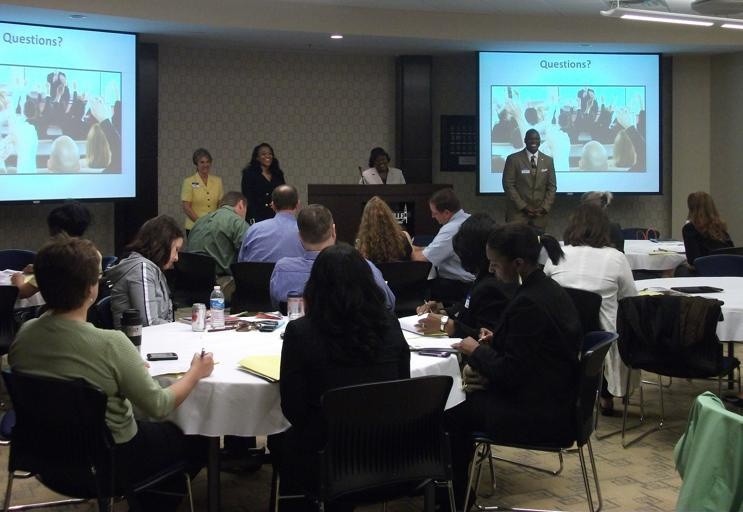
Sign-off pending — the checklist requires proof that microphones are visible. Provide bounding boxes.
[358,165,368,184]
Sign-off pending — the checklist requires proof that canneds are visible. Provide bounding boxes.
[191,303,206,332]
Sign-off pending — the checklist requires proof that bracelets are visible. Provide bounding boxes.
[440,315,449,333]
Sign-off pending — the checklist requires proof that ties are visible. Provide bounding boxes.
[530,155,539,177]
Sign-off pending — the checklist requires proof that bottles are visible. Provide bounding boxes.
[209,286,225,330]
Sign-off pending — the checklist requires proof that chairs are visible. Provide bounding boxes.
[616,295,740,449]
[374,260,433,317]
[230,262,277,312]
[462,332,618,510]
[275,376,456,511]
[2,249,119,330]
[683,247,741,277]
[1,371,193,510]
[164,252,219,309]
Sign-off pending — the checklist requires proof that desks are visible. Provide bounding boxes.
[129,316,462,509]
[634,277,740,390]
[539,239,689,270]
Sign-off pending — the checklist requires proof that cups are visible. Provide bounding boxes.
[287,291,305,321]
[119,309,143,354]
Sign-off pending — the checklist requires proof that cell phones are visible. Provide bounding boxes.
[146,352,180,360]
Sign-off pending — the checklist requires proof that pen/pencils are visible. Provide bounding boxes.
[208,327,237,331]
[201,348,205,359]
[424,299,434,314]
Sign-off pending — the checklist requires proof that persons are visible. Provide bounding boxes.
[267,243,434,512]
[270,205,397,317]
[181,191,271,309]
[358,146,407,185]
[4,236,213,509]
[543,206,637,413]
[405,189,492,311]
[501,129,557,234]
[0,204,102,446]
[446,223,584,504]
[180,148,225,239]
[490,84,645,170]
[241,141,285,225]
[354,197,412,265]
[674,190,735,275]
[237,187,303,265]
[104,213,185,331]
[1,69,121,174]
